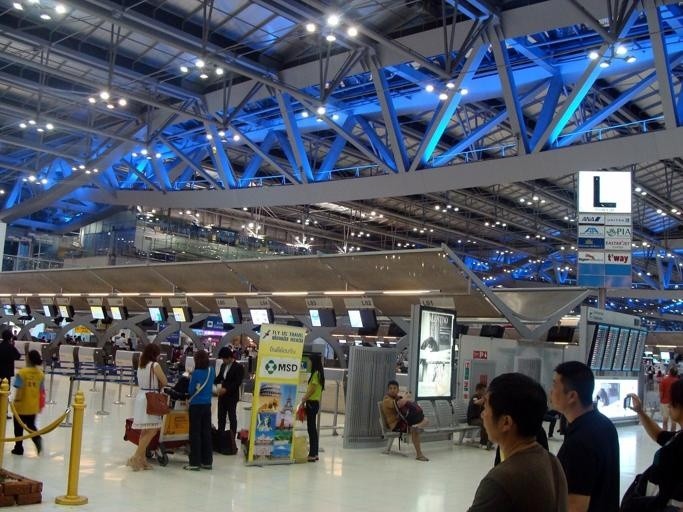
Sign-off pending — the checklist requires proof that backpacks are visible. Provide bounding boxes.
[392,396,424,426]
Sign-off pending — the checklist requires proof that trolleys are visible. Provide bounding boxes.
[122,390,189,466]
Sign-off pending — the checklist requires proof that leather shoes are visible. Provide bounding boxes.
[200,463,211,469]
[11,448,22,454]
[183,465,200,471]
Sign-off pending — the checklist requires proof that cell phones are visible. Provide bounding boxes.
[624,396,631,409]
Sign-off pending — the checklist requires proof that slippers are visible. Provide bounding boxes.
[416,456,429,461]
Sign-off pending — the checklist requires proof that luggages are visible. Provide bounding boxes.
[123,418,185,450]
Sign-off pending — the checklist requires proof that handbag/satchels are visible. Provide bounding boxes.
[212,429,236,454]
[145,361,168,415]
[620,473,668,512]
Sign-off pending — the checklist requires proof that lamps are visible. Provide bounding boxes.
[300,105,339,124]
[587,42,636,69]
[205,127,241,145]
[423,82,469,102]
[178,57,224,81]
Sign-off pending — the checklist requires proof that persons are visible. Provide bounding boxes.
[466,383,488,449]
[627,377,682,464]
[0,329,257,471]
[465,372,569,512]
[548,361,619,511]
[302,355,325,461]
[382,379,429,461]
[542,409,568,436]
[657,368,678,432]
[644,353,683,380]
[418,336,450,380]
[493,424,547,467]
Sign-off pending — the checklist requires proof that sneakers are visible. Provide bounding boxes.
[478,443,487,449]
[307,456,319,461]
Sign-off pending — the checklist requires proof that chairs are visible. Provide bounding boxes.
[376,397,481,456]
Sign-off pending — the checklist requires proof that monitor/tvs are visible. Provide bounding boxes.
[41,304,58,317]
[347,308,576,343]
[148,306,169,322]
[16,304,31,318]
[110,305,129,320]
[2,304,16,317]
[249,308,275,326]
[219,307,243,324]
[90,306,108,320]
[58,304,75,318]
[172,306,193,322]
[308,308,337,327]
